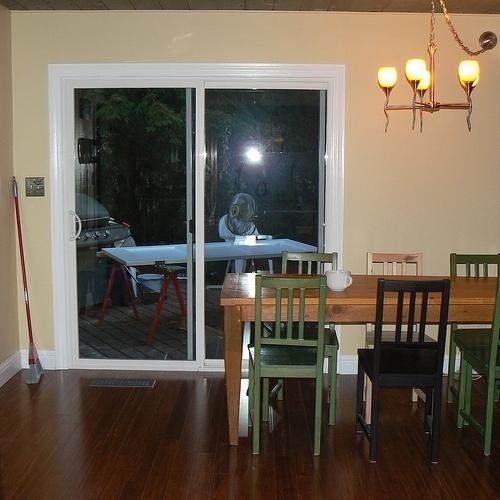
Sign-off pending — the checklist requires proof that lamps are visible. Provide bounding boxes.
[377,1,496,132]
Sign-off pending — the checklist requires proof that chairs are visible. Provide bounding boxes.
[260,250,342,426]
[247,273,328,458]
[456,278,500,456]
[362,250,437,426]
[446,252,499,430]
[354,275,452,462]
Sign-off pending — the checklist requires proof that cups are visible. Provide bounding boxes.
[325,270,353,291]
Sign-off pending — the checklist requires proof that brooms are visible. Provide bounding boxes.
[11,176,44,384]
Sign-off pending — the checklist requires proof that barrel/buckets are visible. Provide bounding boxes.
[137,273,162,292]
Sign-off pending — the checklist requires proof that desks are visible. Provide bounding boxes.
[220,272,500,445]
[98,238,318,345]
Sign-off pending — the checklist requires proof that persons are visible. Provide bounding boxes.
[218,193,260,273]
